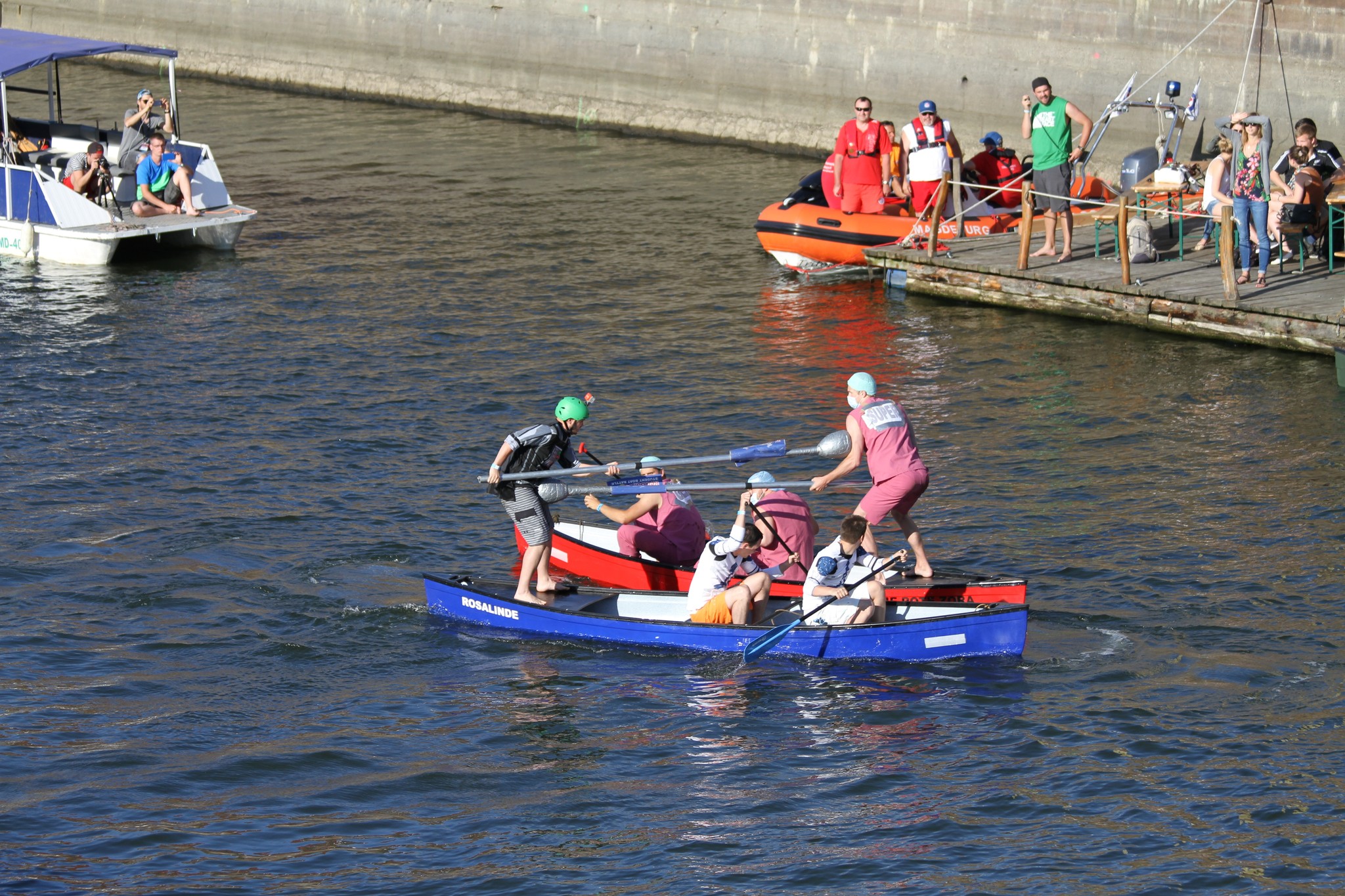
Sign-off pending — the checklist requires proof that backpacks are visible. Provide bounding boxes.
[1126,215,1156,263]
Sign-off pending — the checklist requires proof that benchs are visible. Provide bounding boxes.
[1090,187,1150,263]
[1214,205,1330,275]
[20,150,137,201]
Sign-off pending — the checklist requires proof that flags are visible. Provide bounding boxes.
[1110,76,1200,122]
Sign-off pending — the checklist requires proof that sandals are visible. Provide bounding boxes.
[1236,276,1251,285]
[1255,277,1266,287]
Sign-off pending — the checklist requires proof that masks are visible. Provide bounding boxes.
[847,394,862,408]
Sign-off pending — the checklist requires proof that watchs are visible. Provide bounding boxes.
[1077,145,1085,152]
[180,163,184,167]
[882,181,890,185]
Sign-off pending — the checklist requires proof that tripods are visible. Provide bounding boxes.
[97,172,122,217]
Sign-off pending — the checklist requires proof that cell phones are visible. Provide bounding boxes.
[152,100,162,107]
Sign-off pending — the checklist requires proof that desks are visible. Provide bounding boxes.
[1130,161,1211,262]
[1325,178,1345,275]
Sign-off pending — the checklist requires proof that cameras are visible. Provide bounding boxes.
[584,393,595,404]
[97,158,110,170]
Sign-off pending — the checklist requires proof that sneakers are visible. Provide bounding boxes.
[1255,240,1279,254]
[1269,233,1275,241]
[1302,236,1319,259]
[1271,250,1293,264]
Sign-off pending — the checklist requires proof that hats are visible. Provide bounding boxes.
[1032,77,1050,92]
[137,89,152,108]
[748,471,786,491]
[979,131,1003,146]
[87,142,104,154]
[847,372,876,395]
[639,456,663,471]
[918,100,936,114]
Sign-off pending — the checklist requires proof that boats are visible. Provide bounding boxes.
[418,514,1030,659]
[0,27,257,269]
[753,164,1206,277]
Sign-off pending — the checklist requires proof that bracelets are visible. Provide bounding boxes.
[490,463,501,471]
[596,503,604,514]
[890,554,899,564]
[1277,195,1281,202]
[165,110,170,113]
[736,510,746,516]
[1023,109,1030,113]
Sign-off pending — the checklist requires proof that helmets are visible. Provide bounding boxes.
[555,396,589,422]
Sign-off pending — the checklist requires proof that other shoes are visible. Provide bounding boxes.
[1194,241,1206,250]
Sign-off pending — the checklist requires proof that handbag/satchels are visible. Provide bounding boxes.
[1280,203,1319,225]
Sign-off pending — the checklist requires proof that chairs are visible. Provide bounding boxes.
[1021,155,1034,181]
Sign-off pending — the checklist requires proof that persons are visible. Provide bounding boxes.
[59,88,204,219]
[799,514,908,626]
[818,96,1345,289]
[584,456,706,567]
[1019,76,1093,262]
[482,397,620,605]
[731,470,819,582]
[688,490,801,624]
[807,372,934,586]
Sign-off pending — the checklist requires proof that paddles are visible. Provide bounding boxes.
[578,443,710,539]
[745,502,808,575]
[743,550,904,662]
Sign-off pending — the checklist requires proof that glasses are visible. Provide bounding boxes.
[1233,129,1243,133]
[1245,123,1257,127]
[140,99,148,105]
[855,107,870,111]
[922,113,935,116]
